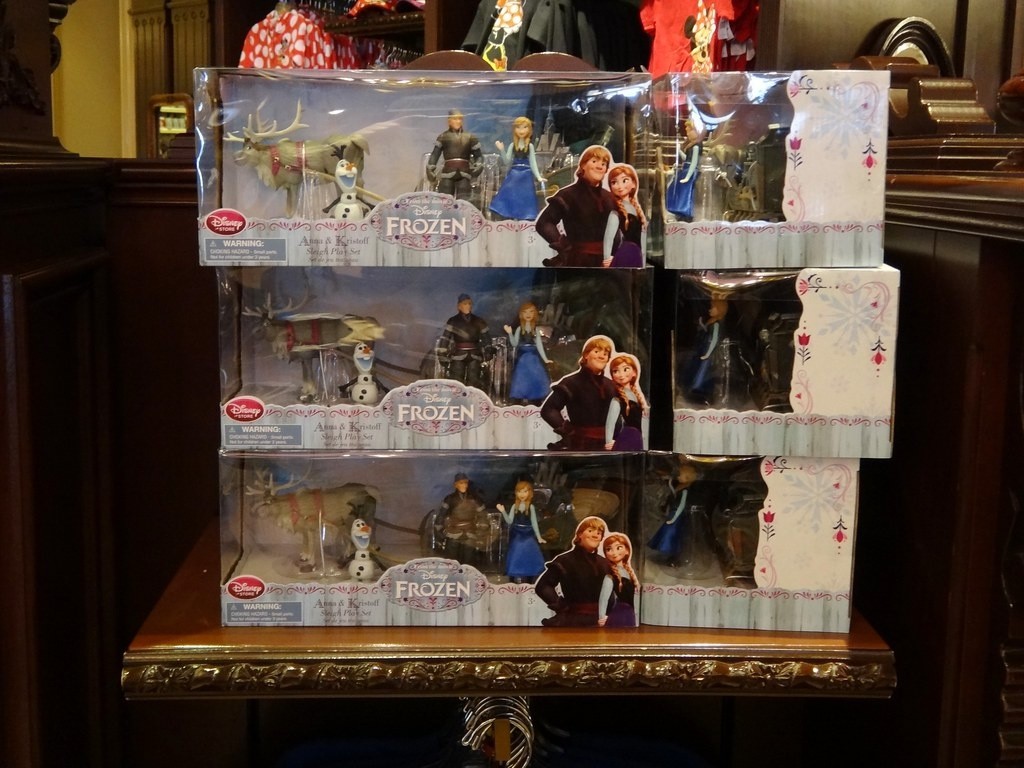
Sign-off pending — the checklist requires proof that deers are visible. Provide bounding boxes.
[242,285,387,406]
[221,95,371,218]
[244,460,381,573]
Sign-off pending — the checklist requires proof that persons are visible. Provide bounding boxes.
[687,297,728,409]
[485,116,549,222]
[643,465,699,568]
[501,301,555,408]
[434,471,492,568]
[753,328,782,396]
[664,113,707,221]
[496,480,550,585]
[735,146,759,189]
[434,292,498,400]
[426,109,484,204]
